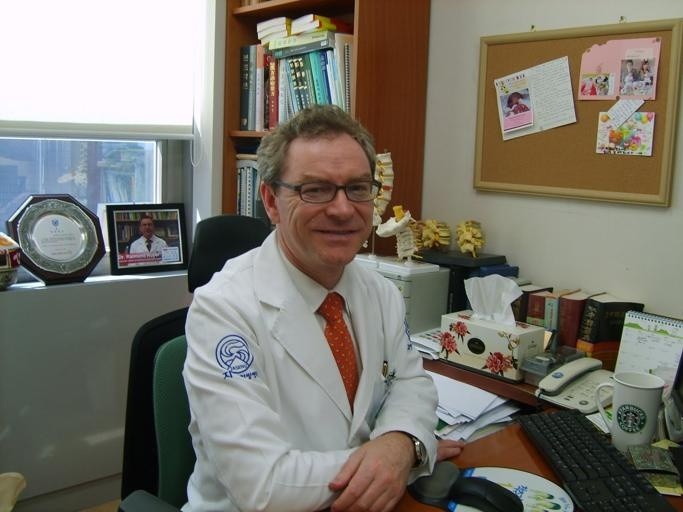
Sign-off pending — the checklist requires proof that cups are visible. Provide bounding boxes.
[596,373,668,456]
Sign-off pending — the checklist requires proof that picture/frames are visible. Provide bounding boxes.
[106,200,188,279]
[472,18,675,211]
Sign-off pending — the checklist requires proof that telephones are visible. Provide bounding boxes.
[535,358,614,415]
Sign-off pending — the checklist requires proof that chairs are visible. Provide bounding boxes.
[114,213,273,511]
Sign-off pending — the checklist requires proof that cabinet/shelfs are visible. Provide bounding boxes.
[220,0,434,255]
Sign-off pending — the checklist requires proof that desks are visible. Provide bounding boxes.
[385,365,683,511]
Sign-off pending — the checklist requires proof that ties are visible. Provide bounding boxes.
[145,240,153,253]
[316,292,360,413]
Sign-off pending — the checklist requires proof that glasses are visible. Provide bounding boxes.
[273,178,382,204]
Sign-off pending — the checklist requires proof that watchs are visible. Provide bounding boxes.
[408,433,427,469]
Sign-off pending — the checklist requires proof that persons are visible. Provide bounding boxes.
[636,58,654,82]
[126,215,168,267]
[181,101,465,511]
[621,59,640,85]
[506,92,530,116]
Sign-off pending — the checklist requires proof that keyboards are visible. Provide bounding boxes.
[521,408,677,511]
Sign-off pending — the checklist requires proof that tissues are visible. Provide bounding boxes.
[439,272,546,383]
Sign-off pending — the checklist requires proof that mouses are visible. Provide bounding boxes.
[450,477,523,511]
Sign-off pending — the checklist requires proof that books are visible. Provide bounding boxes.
[510,284,553,323]
[574,291,644,373]
[558,288,609,349]
[544,287,581,328]
[526,290,558,327]
[234,0,354,224]
